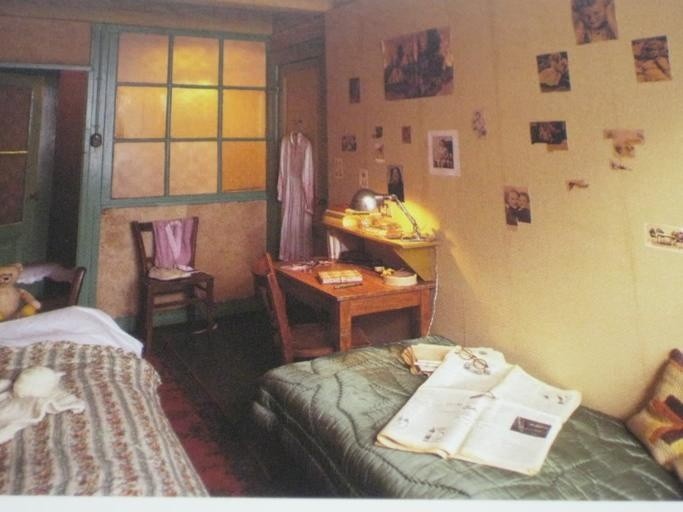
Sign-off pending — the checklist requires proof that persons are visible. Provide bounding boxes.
[515,192,531,224]
[640,37,671,82]
[438,139,449,168]
[540,52,565,86]
[574,0,618,44]
[507,189,520,225]
[388,167,405,203]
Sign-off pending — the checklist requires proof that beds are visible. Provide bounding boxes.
[0,305,216,497]
[249,335,683,501]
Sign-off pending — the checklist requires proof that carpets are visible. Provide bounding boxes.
[127,331,289,497]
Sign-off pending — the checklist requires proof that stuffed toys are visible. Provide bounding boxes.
[0,262,41,323]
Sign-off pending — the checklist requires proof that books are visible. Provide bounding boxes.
[318,270,363,284]
[374,343,582,475]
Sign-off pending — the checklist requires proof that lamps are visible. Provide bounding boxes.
[349,189,427,241]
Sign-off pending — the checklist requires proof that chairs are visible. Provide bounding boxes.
[131,216,215,355]
[10,260,86,320]
[250,253,365,366]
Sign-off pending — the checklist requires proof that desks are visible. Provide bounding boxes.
[270,204,438,352]
